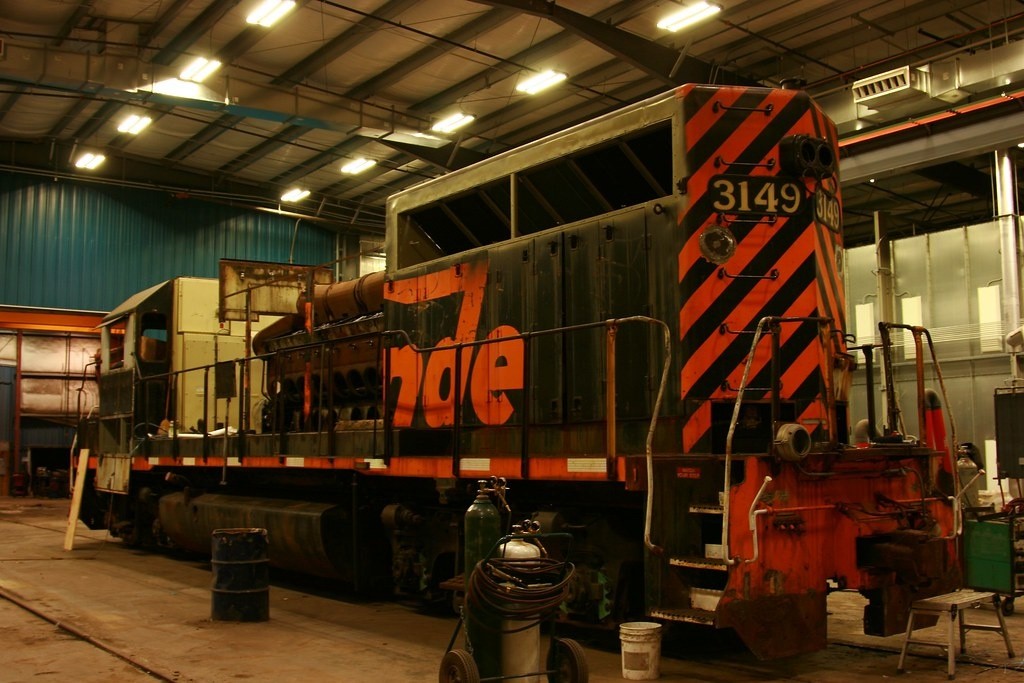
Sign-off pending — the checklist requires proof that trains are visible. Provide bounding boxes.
[69,82,985,663]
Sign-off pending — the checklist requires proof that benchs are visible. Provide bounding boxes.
[895,590,1015,679]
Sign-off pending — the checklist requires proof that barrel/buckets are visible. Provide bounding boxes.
[619,621,662,680]
[210,528,272,623]
